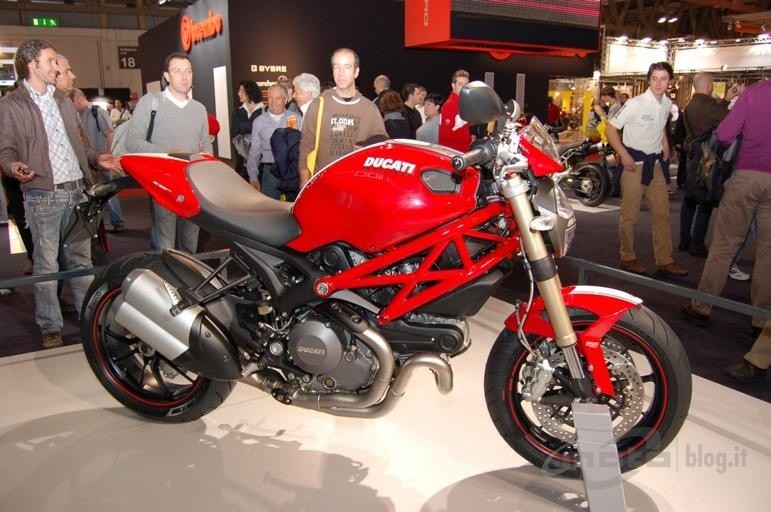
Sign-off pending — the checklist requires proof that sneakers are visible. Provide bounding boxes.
[115,225,124,232]
[682,304,710,327]
[725,361,764,383]
[728,267,750,281]
[42,333,62,348]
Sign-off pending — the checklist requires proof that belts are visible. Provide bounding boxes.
[57,178,86,189]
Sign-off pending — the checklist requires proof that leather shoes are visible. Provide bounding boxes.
[619,259,647,272]
[657,263,688,276]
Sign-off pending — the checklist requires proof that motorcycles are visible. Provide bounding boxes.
[61,80,694,481]
[504,100,616,207]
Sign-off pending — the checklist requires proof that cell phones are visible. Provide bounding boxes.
[19,167,31,175]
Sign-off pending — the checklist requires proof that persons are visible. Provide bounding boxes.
[760,23,771,38]
[0,38,476,349]
[548,62,771,386]
